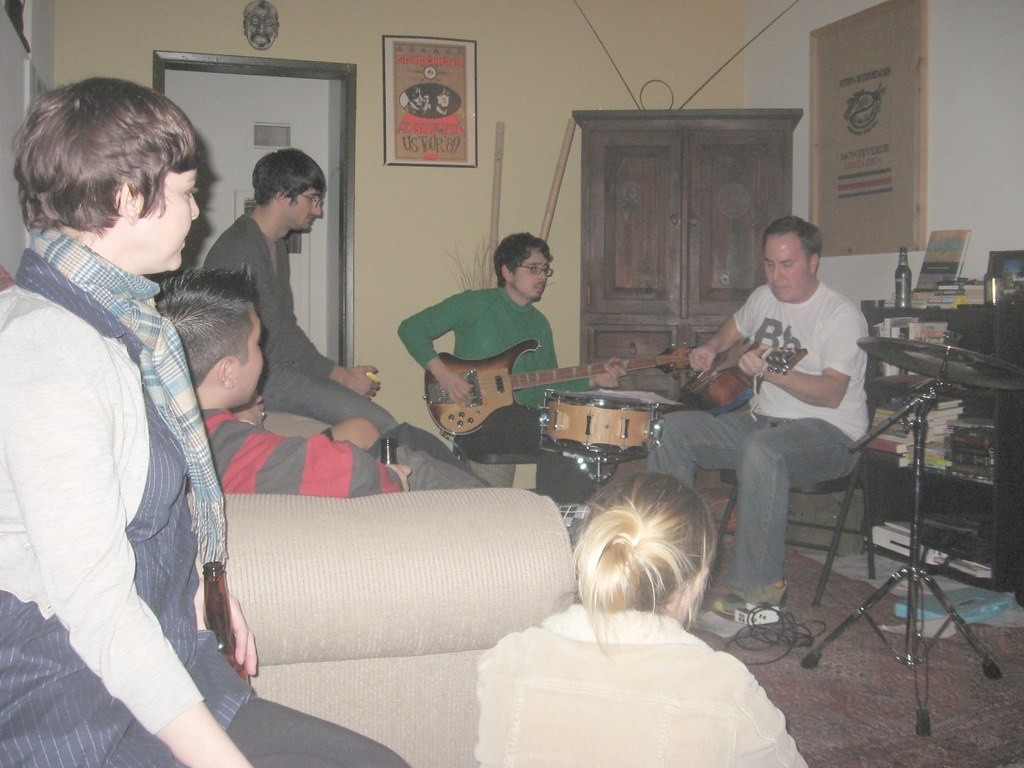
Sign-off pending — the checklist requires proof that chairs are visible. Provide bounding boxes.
[453,442,538,491]
[711,321,878,606]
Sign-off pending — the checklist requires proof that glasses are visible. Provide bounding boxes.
[298,192,325,210]
[509,265,554,278]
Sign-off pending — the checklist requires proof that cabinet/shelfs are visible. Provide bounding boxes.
[571,110,804,488]
[859,281,1024,595]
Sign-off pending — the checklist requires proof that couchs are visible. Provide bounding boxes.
[172,411,573,768]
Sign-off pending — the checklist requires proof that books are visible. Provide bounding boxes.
[866,394,993,484]
[873,316,947,376]
[911,229,985,309]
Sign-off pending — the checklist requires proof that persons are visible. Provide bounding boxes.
[203,146,398,432]
[477,472,808,768]
[646,216,873,618]
[0,77,409,768]
[151,263,591,543]
[397,231,626,506]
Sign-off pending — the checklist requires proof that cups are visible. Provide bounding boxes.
[983,273,1003,304]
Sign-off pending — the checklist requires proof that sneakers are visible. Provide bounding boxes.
[713,589,788,615]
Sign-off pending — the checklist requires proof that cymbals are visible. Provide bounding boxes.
[856,336,1024,390]
[870,375,969,412]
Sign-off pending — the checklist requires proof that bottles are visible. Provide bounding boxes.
[202,562,248,686]
[379,439,398,466]
[895,247,911,310]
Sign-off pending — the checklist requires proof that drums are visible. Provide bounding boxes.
[539,388,660,465]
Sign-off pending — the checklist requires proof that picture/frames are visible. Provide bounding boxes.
[382,36,479,169]
[985,250,1024,305]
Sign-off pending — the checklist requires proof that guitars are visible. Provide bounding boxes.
[684,338,808,414]
[423,338,693,436]
